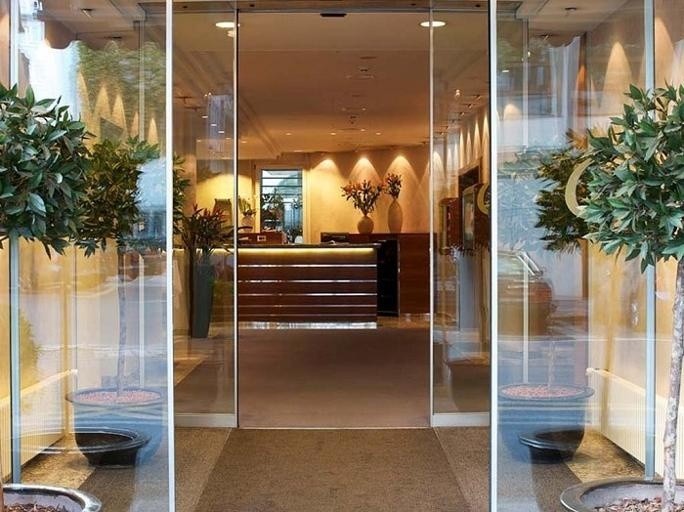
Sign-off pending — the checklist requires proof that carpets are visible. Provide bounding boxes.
[67,423,585,511]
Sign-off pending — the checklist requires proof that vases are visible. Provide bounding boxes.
[358,198,404,235]
[443,354,491,409]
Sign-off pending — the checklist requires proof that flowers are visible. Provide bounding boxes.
[340,172,403,217]
